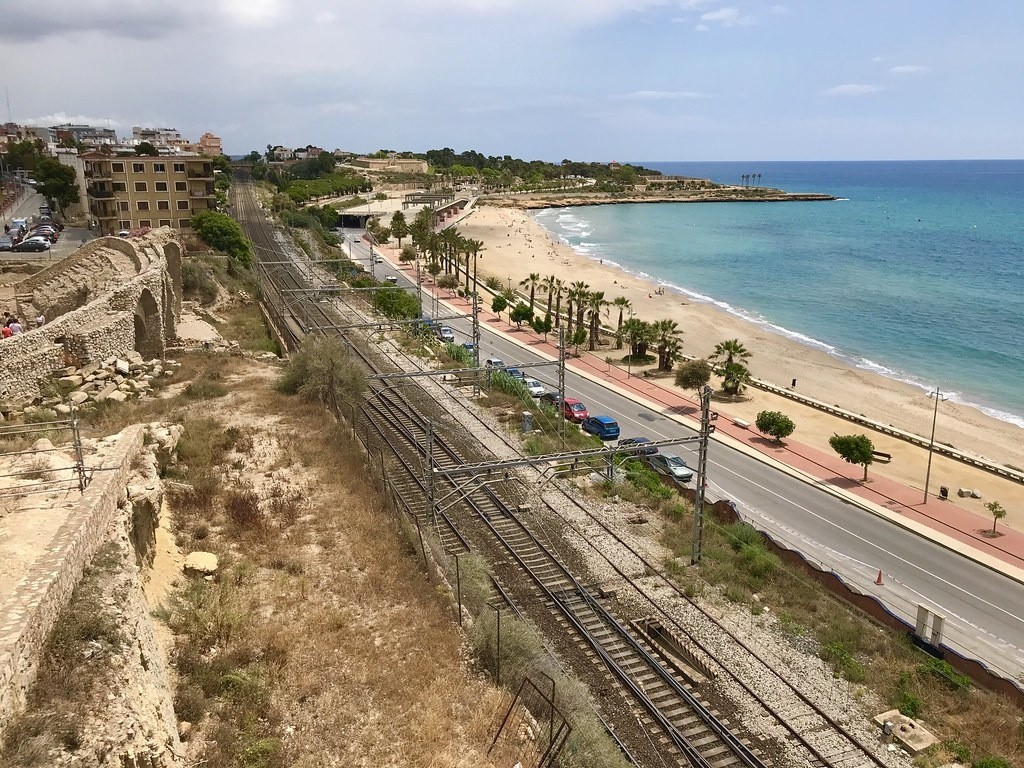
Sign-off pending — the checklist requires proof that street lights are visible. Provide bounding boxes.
[924,387,949,506]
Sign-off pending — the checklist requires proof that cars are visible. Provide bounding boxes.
[421,320,455,343]
[558,397,590,424]
[461,341,475,357]
[384,274,397,283]
[486,359,507,370]
[503,367,524,383]
[540,391,566,412]
[618,437,659,460]
[0,175,65,252]
[518,376,546,398]
[650,450,694,482]
[119,229,129,237]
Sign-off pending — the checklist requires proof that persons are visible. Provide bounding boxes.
[0,312,24,339]
[599,258,603,264]
[647,285,666,298]
[3,223,10,233]
[35,311,46,327]
[17,224,25,239]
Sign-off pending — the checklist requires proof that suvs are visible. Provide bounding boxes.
[582,415,620,440]
